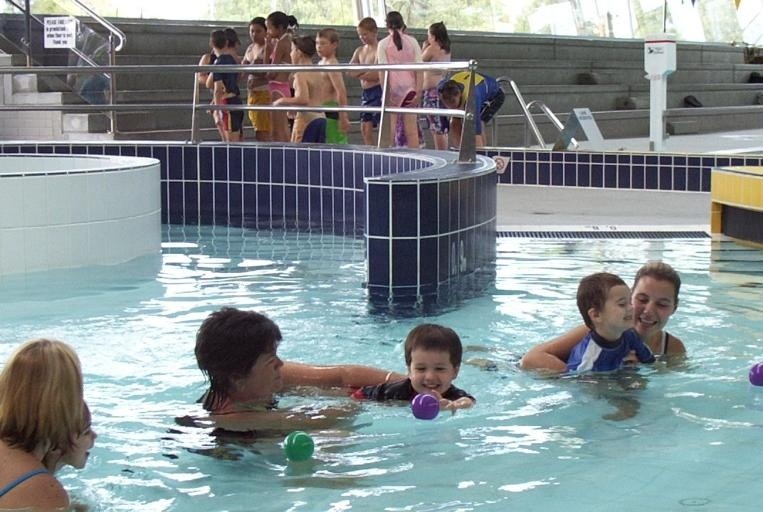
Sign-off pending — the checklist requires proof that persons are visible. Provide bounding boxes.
[421,20,505,149]
[0,338,84,512]
[345,11,424,148]
[564,273,657,372]
[240,12,351,144]
[194,305,409,429]
[196,29,245,144]
[43,400,97,471]
[518,262,686,373]
[349,323,476,415]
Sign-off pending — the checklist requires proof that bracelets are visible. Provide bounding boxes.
[385,370,394,382]
